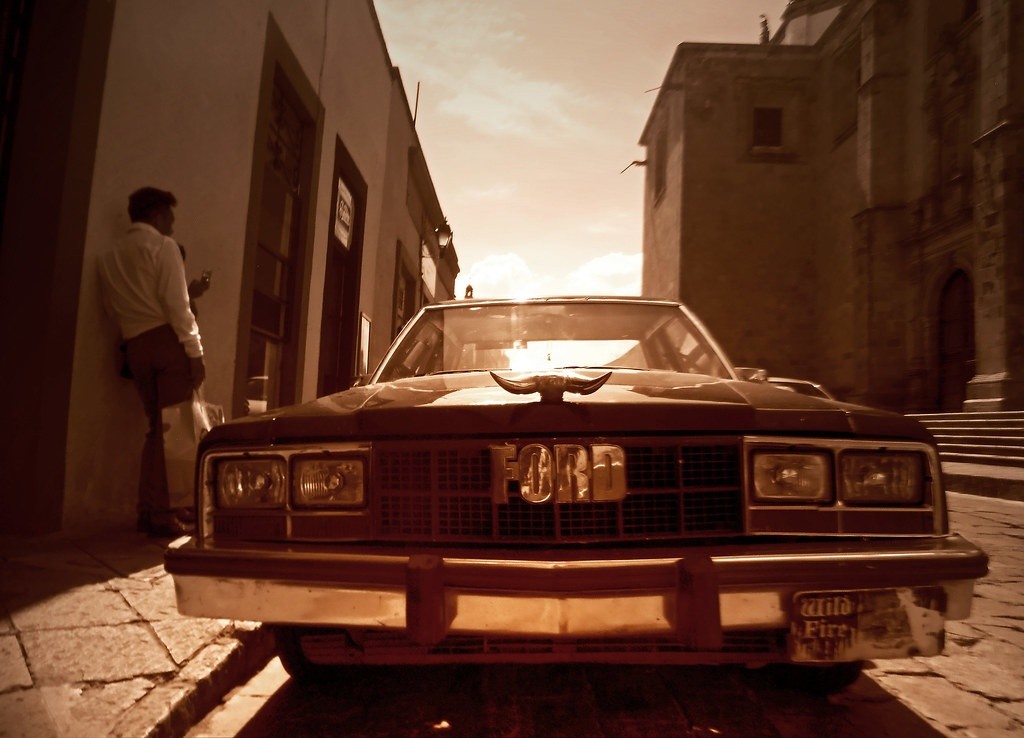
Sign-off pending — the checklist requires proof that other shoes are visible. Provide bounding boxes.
[176,507,196,522]
[135,515,196,536]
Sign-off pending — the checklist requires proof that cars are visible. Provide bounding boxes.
[163,297,990,700]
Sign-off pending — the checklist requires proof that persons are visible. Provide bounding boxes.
[95,188,205,540]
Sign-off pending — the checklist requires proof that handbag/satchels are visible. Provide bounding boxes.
[161,383,224,510]
